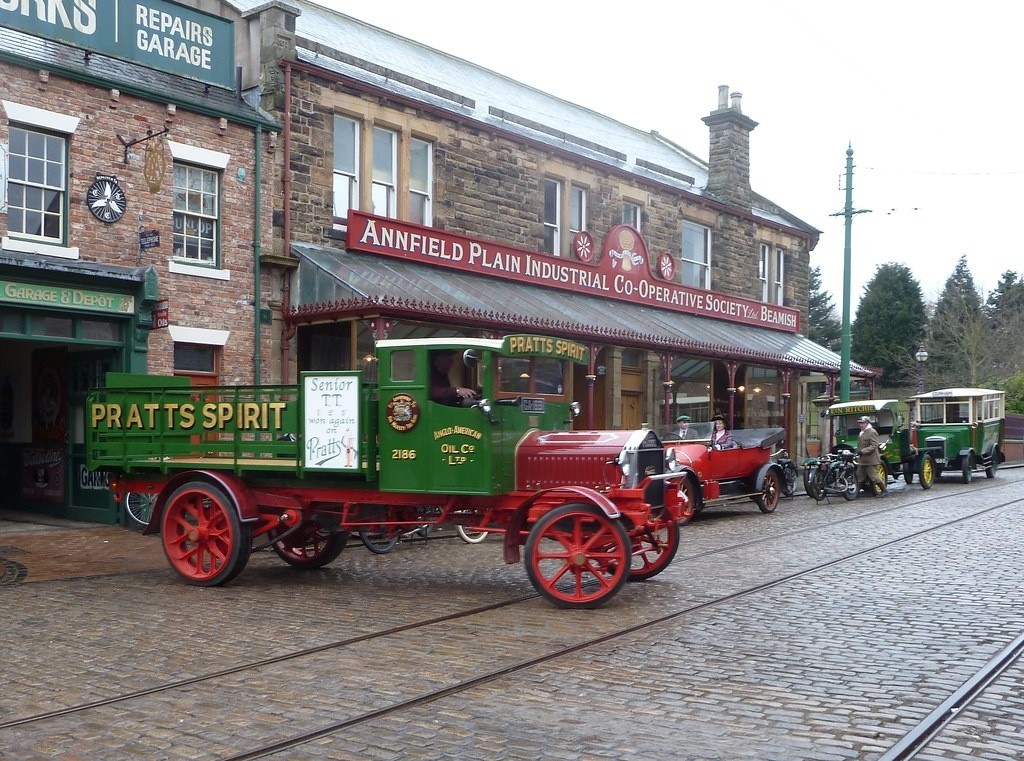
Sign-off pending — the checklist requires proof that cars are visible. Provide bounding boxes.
[653,420,787,521]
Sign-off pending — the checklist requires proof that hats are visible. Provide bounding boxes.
[430,350,458,356]
[711,414,729,425]
[857,416,871,423]
[676,415,691,421]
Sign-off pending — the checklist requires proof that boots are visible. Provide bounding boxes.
[856,482,863,491]
[876,482,889,497]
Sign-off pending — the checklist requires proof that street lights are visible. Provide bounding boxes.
[916,341,929,396]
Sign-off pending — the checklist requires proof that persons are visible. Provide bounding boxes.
[669,415,699,439]
[427,349,478,407]
[854,416,890,498]
[709,414,733,450]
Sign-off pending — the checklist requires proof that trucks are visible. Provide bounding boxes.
[85,334,688,610]
[911,387,1007,484]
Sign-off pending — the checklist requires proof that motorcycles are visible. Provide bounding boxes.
[801,398,920,503]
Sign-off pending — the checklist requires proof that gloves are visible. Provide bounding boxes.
[858,450,863,456]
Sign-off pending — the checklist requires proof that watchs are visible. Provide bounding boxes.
[456,387,460,396]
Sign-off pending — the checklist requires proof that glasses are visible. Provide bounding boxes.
[683,422,691,423]
[858,417,866,420]
